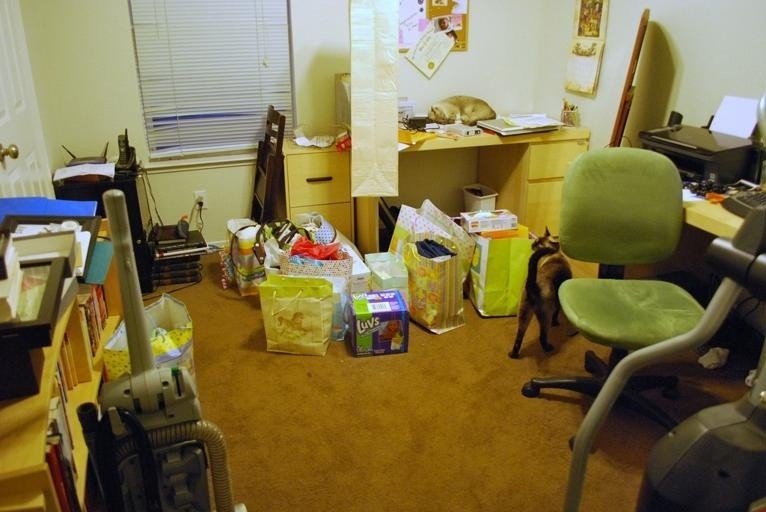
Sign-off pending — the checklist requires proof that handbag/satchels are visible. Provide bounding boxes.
[392,215,467,336]
[101,292,196,392]
[388,198,475,314]
[227,197,408,357]
[456,209,538,317]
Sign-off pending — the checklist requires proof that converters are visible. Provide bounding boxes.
[408,117,426,129]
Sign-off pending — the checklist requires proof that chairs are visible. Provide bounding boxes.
[521,146,709,450]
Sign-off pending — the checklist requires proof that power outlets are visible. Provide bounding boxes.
[0,217,125,511]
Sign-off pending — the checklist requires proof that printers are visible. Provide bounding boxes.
[638,111,766,187]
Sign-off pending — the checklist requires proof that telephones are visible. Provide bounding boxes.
[115,127,137,173]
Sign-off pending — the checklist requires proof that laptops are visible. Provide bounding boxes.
[157,230,207,250]
[476,117,563,136]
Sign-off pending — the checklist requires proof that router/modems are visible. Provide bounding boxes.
[61,142,110,166]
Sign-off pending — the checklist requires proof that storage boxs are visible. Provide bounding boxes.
[340,242,371,293]
[350,290,408,358]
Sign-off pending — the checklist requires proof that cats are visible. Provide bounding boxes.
[427,96,497,127]
[508,225,573,360]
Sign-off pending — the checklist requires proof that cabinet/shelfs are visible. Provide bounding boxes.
[282,137,355,245]
[53,167,155,291]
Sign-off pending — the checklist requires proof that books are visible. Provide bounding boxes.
[149,255,202,285]
[44,282,111,510]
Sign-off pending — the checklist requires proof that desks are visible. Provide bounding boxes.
[356,123,591,255]
[680,180,741,239]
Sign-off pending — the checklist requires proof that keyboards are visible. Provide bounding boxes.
[721,192,766,219]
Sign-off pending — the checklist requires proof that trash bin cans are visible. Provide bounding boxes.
[462,184,499,212]
